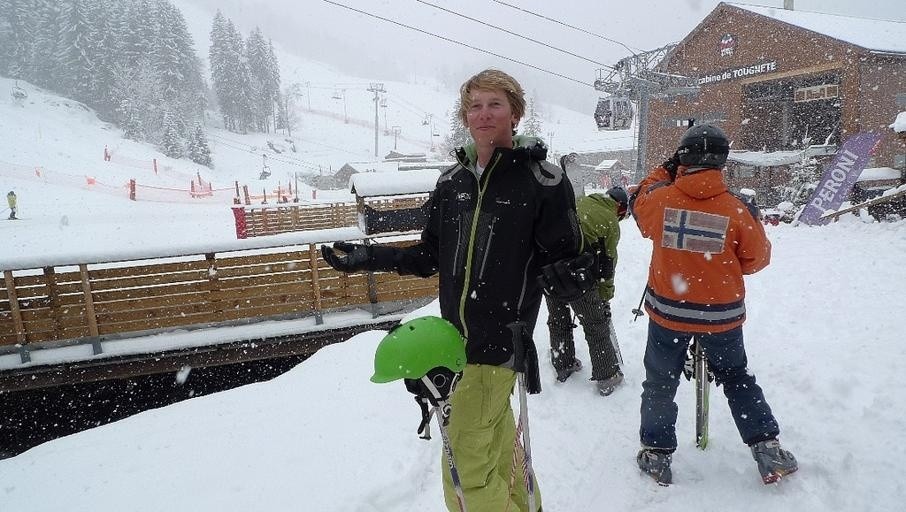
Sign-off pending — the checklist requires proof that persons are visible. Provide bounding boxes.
[630,123,797,487]
[544,186,631,396]
[321,69,598,512]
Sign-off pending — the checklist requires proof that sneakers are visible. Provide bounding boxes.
[556,358,582,381]
[749,438,798,483]
[598,371,625,396]
[637,446,676,484]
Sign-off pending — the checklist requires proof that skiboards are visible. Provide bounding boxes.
[695,338,709,448]
[559,153,624,368]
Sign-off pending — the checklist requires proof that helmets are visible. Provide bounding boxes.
[677,124,729,171]
[605,185,628,222]
[369,316,467,400]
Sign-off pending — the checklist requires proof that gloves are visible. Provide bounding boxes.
[319,238,374,275]
[535,251,593,302]
